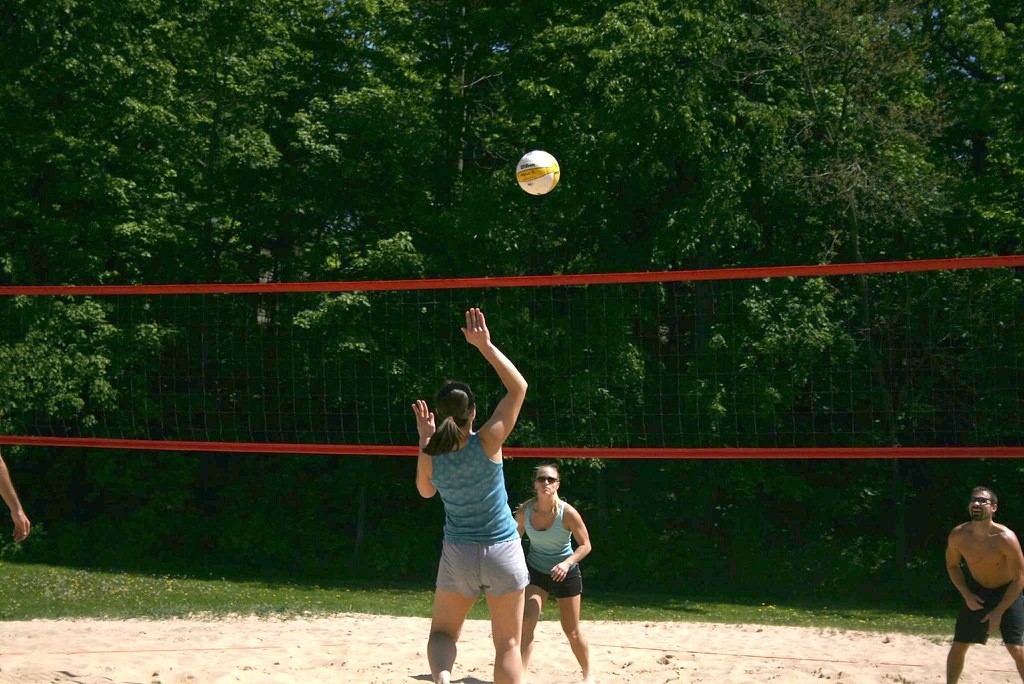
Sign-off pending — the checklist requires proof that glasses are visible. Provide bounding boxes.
[970,496,996,504]
[535,476,559,483]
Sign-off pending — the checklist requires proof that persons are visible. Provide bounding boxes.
[946,486,1024,684]
[0,456,32,542]
[411,307,531,684]
[516,463,594,684]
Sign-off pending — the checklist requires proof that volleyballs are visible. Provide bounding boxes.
[515,149,561,197]
